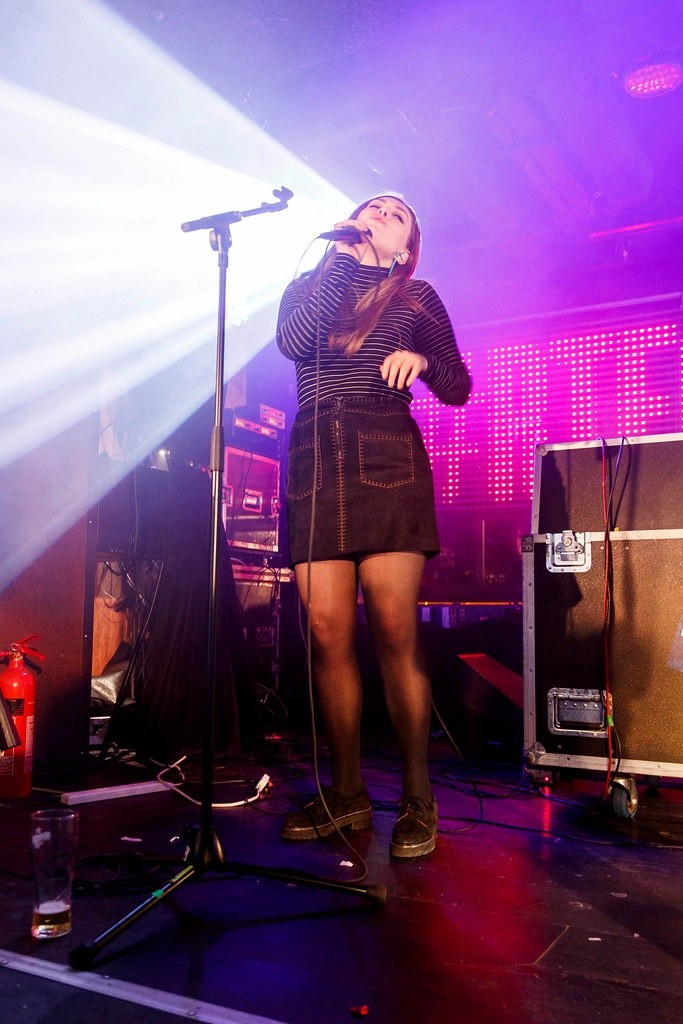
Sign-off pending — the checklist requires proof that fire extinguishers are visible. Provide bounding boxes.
[0,633,47,799]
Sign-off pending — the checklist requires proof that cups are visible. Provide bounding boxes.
[31,810,79,939]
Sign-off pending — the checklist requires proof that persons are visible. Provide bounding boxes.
[276,190,475,857]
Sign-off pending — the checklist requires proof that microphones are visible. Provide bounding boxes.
[320,227,372,244]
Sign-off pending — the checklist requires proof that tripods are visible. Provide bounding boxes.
[65,187,387,971]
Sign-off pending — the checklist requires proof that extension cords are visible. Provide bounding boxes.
[61,781,172,805]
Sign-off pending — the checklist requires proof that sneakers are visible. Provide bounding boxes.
[389,793,437,857]
[281,787,372,839]
